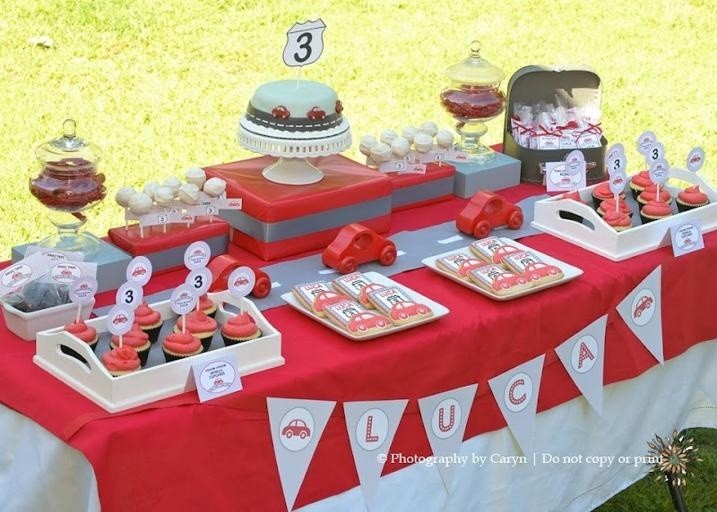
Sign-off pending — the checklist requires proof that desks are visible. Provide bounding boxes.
[0,144,717,512]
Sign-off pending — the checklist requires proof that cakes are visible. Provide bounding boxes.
[560,169,709,233]
[238,77,351,140]
[61,292,262,378]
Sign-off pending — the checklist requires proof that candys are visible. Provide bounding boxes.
[114,167,226,239]
[359,123,454,176]
